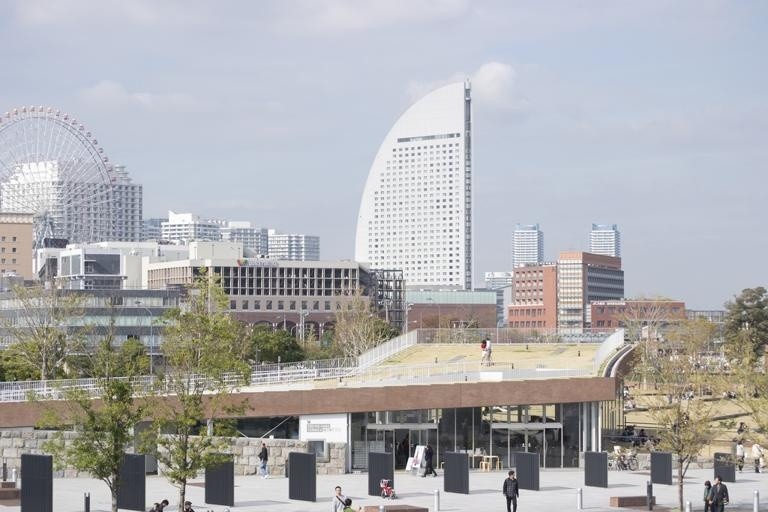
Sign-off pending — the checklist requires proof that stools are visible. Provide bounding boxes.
[479,455,504,472]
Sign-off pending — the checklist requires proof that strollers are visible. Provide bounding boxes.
[378,478,397,498]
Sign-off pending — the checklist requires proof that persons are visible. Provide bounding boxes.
[675,389,694,400]
[722,391,735,399]
[629,397,636,409]
[183,500,195,512]
[155,499,168,512]
[258,442,269,479]
[751,439,762,474]
[502,470,519,511]
[735,440,745,472]
[638,429,647,440]
[623,388,628,398]
[707,475,729,511]
[621,428,630,443]
[421,444,438,477]
[703,479,714,512]
[624,398,632,409]
[341,498,356,512]
[332,485,345,512]
[149,503,159,512]
[484,337,491,367]
[480,340,494,366]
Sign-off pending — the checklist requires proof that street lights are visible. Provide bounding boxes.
[496,318,507,344]
[135,300,155,391]
[425,296,442,342]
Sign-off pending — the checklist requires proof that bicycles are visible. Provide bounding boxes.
[615,455,639,471]
